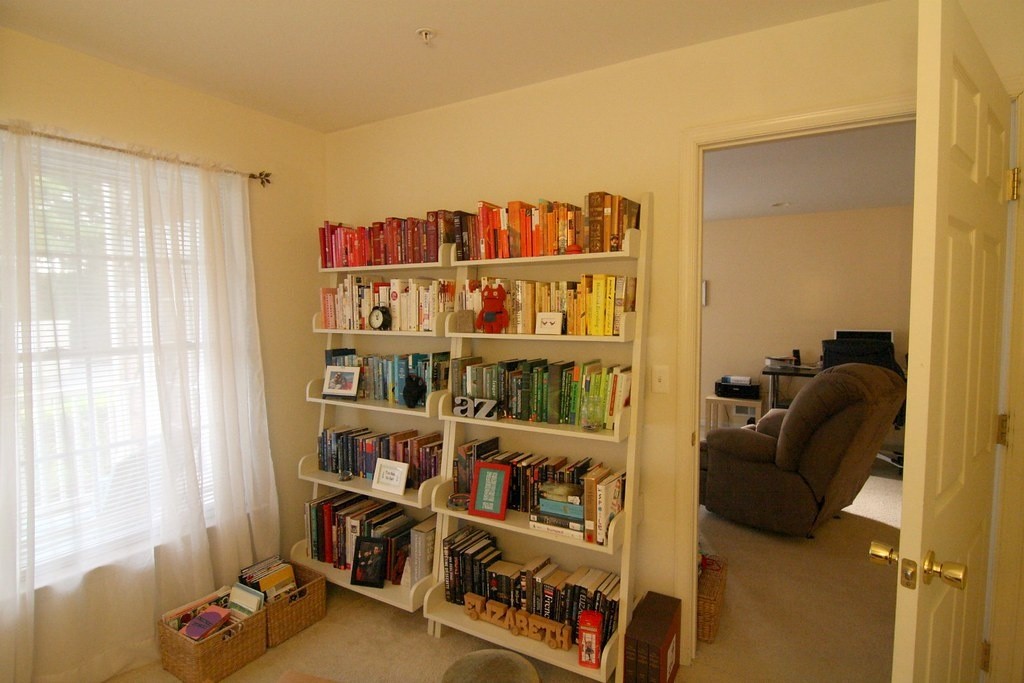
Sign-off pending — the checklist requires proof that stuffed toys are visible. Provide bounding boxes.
[476,284,510,334]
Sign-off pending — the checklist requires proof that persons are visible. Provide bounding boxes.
[340,376,353,390]
[358,549,364,560]
[359,549,370,569]
[367,546,382,579]
[330,373,341,389]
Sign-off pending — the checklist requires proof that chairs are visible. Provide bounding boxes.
[822,340,903,474]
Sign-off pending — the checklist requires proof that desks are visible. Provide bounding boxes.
[762,366,824,408]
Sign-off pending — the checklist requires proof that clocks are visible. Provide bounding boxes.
[369,301,392,331]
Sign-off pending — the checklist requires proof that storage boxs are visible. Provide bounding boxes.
[625,591,682,683]
[579,611,603,668]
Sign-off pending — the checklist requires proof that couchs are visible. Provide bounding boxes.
[698,363,906,539]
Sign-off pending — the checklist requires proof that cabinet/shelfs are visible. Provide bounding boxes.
[291,238,470,637]
[424,192,654,683]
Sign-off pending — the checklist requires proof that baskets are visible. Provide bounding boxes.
[157,605,266,683]
[262,557,326,648]
[696,554,728,644]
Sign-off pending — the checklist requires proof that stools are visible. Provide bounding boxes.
[441,647,539,683]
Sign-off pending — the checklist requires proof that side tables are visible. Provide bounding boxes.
[705,395,763,433]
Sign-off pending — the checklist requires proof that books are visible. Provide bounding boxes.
[161,582,265,642]
[238,556,300,603]
[324,347,632,431]
[320,274,636,336]
[317,424,627,547]
[303,490,620,652]
[721,375,751,385]
[318,192,641,268]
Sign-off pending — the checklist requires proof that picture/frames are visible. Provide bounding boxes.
[350,536,390,588]
[467,461,512,520]
[535,312,562,335]
[321,365,361,401]
